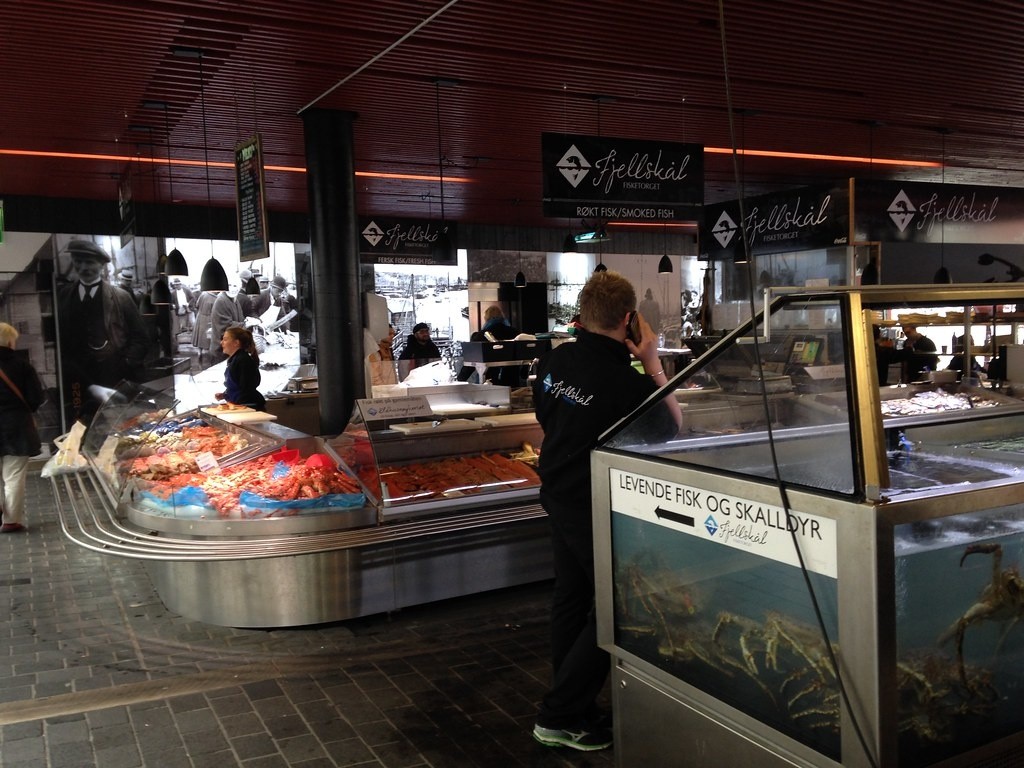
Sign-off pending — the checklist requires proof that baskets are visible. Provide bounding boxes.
[249,324,267,353]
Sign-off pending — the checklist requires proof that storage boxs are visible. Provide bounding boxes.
[461,337,577,364]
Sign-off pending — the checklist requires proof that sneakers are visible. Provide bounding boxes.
[585,705,612,728]
[533,721,612,750]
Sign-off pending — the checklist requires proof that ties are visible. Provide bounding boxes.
[83,285,91,307]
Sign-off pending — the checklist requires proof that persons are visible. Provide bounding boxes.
[170,272,296,356]
[531,272,682,751]
[116,268,137,301]
[947,335,985,382]
[0,321,48,532]
[398,323,442,383]
[215,326,268,412]
[871,324,937,386]
[43,239,153,414]
[369,324,397,385]
[483,305,511,330]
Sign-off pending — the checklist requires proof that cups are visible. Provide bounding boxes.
[942,346,947,353]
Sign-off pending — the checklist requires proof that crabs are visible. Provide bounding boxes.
[614,539,1024,768]
[115,425,359,519]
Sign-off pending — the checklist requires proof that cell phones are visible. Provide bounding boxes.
[626,311,642,346]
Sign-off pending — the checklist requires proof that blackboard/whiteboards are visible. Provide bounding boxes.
[234,132,270,262]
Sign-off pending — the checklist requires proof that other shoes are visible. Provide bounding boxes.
[191,366,198,371]
[0,524,24,533]
[201,364,209,369]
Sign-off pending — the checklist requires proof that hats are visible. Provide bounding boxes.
[118,270,135,280]
[413,323,427,334]
[260,276,269,282]
[64,241,110,263]
[172,279,181,285]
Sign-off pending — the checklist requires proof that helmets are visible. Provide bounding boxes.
[269,276,286,291]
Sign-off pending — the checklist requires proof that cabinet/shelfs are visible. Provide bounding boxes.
[870,322,1024,356]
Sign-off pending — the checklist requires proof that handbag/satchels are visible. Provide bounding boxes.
[26,413,42,456]
[41,421,89,477]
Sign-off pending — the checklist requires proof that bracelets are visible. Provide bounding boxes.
[651,370,664,377]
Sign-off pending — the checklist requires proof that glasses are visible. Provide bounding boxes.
[416,330,428,334]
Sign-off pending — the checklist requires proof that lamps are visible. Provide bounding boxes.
[513,199,528,290]
[657,220,674,274]
[138,52,261,317]
[428,84,452,262]
[934,130,955,284]
[861,125,879,287]
[735,112,752,265]
[567,100,611,272]
